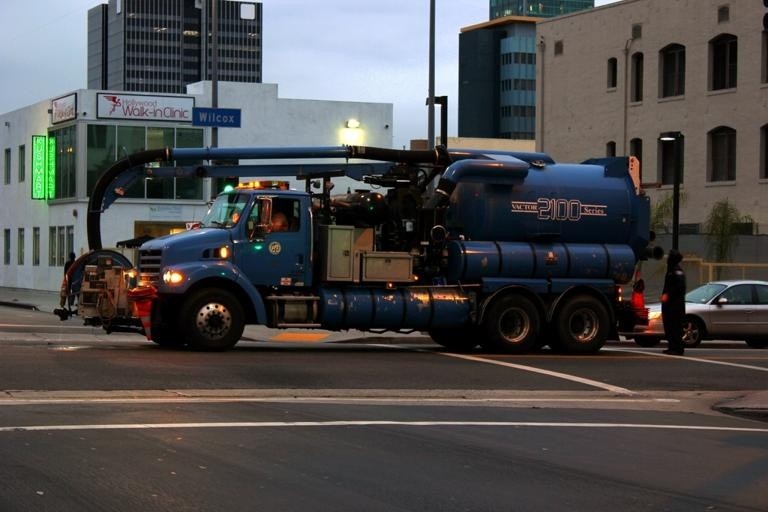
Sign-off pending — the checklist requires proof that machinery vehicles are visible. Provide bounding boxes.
[54,145,666,357]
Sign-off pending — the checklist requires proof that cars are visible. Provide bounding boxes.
[627,279,768,346]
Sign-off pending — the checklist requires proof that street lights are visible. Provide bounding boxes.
[656,131,688,356]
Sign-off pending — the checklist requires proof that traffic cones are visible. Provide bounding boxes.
[129,295,159,341]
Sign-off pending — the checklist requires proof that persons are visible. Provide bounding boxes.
[272,199,288,231]
[64,252,76,307]
[661,249,686,356]
